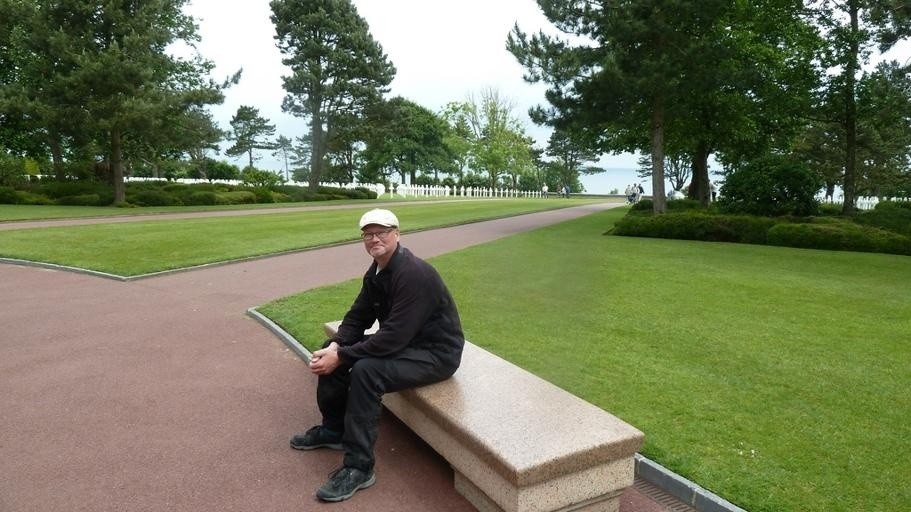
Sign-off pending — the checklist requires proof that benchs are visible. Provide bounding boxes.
[324,316,646,512]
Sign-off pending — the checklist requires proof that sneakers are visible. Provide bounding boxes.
[290,424,344,450]
[316,469,376,503]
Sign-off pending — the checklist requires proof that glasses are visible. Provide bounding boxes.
[360,227,396,241]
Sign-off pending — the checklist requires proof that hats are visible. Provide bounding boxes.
[359,208,399,229]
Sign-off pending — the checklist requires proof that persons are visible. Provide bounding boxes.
[561,185,566,198]
[556,184,561,197]
[286,205,464,505]
[711,180,718,201]
[541,181,548,198]
[624,185,631,203]
[709,179,713,195]
[564,184,570,198]
[630,182,641,203]
[637,184,644,204]
